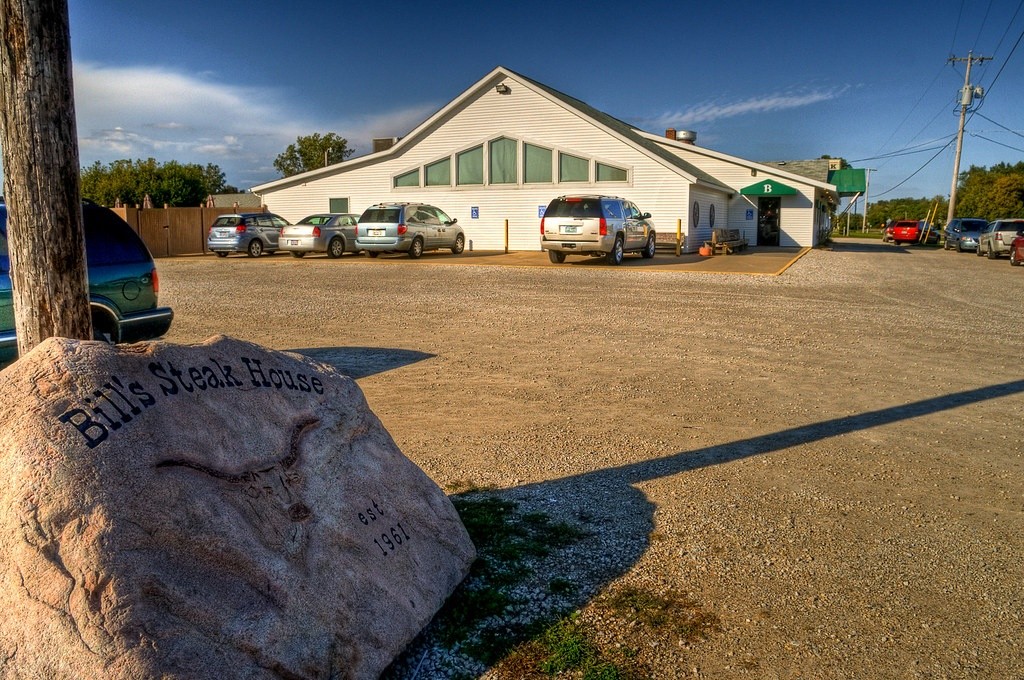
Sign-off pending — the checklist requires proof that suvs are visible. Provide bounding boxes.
[539,194,657,265]
[0,193,175,372]
[207,213,294,258]
[355,201,465,259]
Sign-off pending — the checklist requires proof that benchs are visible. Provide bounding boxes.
[712,228,749,255]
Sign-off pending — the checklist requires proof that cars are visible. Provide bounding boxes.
[881,215,1024,266]
[278,213,362,258]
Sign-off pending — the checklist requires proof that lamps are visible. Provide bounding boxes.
[778,161,785,165]
[751,169,755,176]
[495,83,507,92]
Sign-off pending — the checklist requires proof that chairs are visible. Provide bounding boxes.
[571,208,586,217]
